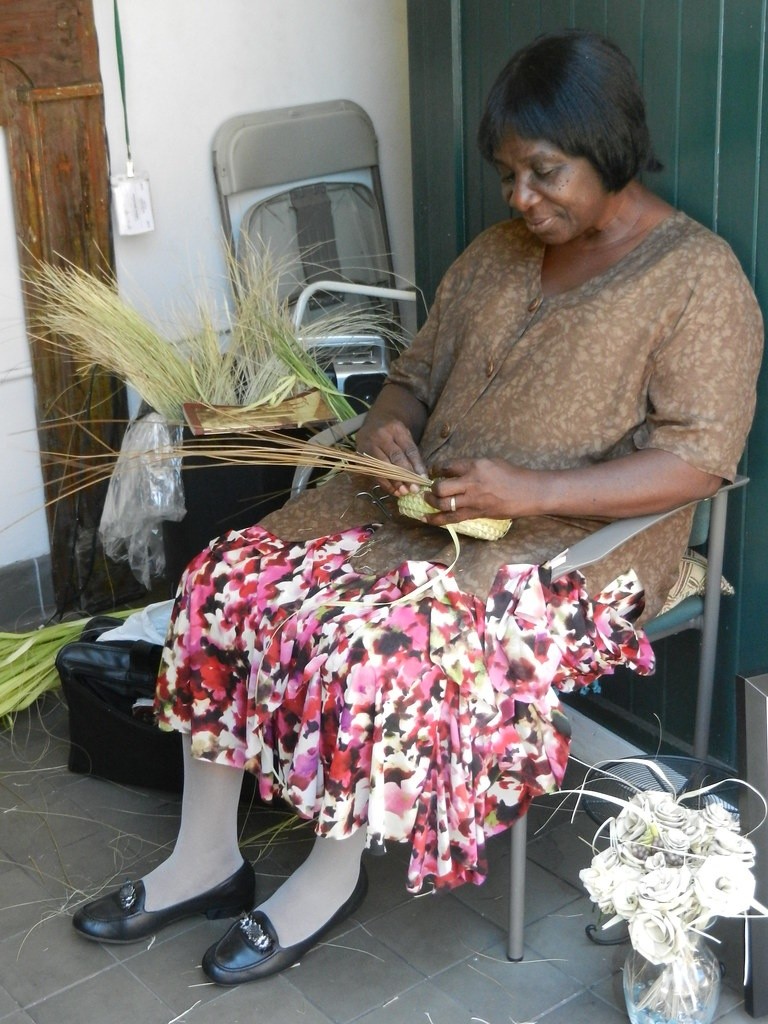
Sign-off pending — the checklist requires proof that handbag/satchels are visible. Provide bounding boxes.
[56,614,258,800]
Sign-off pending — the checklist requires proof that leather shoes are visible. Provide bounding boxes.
[201,860,371,984]
[71,858,256,945]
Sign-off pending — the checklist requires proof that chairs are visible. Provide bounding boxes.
[210,98,426,362]
[286,414,753,959]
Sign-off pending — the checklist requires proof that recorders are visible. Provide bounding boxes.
[271,325,391,415]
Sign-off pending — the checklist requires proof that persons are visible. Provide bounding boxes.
[72,34,765,987]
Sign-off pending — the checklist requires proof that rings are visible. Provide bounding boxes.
[451,497,456,512]
